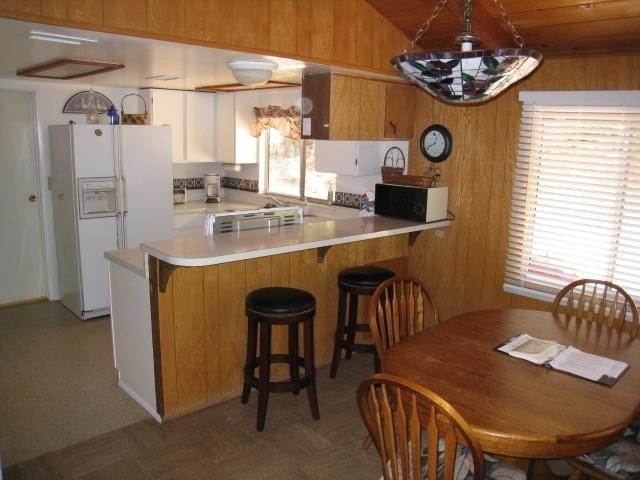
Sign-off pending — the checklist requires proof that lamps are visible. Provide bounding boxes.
[226,57,278,86]
[386,1,543,107]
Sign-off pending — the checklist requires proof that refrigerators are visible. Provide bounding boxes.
[48,123,174,323]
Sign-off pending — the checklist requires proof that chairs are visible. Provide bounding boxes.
[357,373,531,480]
[554,280,640,336]
[567,422,639,480]
[362,276,440,450]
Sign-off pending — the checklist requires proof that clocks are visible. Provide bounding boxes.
[420,125,451,161]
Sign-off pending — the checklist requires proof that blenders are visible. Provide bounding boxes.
[202,173,221,204]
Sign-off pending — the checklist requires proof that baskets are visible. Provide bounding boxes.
[392,174,435,189]
[381,147,405,185]
[121,94,148,126]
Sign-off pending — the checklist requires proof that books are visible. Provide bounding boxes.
[493,332,630,388]
[508,339,558,359]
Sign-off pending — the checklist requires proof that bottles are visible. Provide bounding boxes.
[107,105,120,124]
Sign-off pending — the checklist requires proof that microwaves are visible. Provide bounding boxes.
[373,182,449,223]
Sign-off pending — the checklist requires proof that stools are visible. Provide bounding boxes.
[241,265,397,434]
